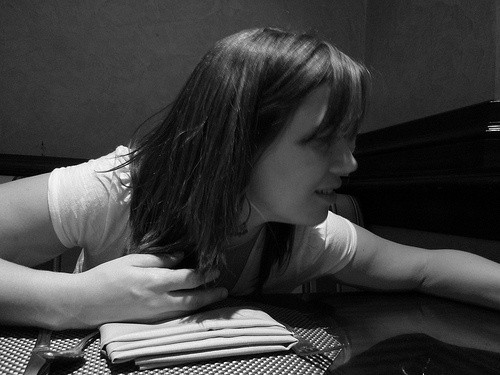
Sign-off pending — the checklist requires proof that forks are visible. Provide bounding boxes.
[270,308,334,372]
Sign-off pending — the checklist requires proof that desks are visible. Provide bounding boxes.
[0,281,351,375]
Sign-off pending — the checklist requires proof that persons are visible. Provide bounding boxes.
[324,296,500,374]
[1,28,500,331]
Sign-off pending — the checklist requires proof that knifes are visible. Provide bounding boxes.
[22,330,53,375]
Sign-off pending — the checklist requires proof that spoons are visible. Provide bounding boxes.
[33,329,100,364]
